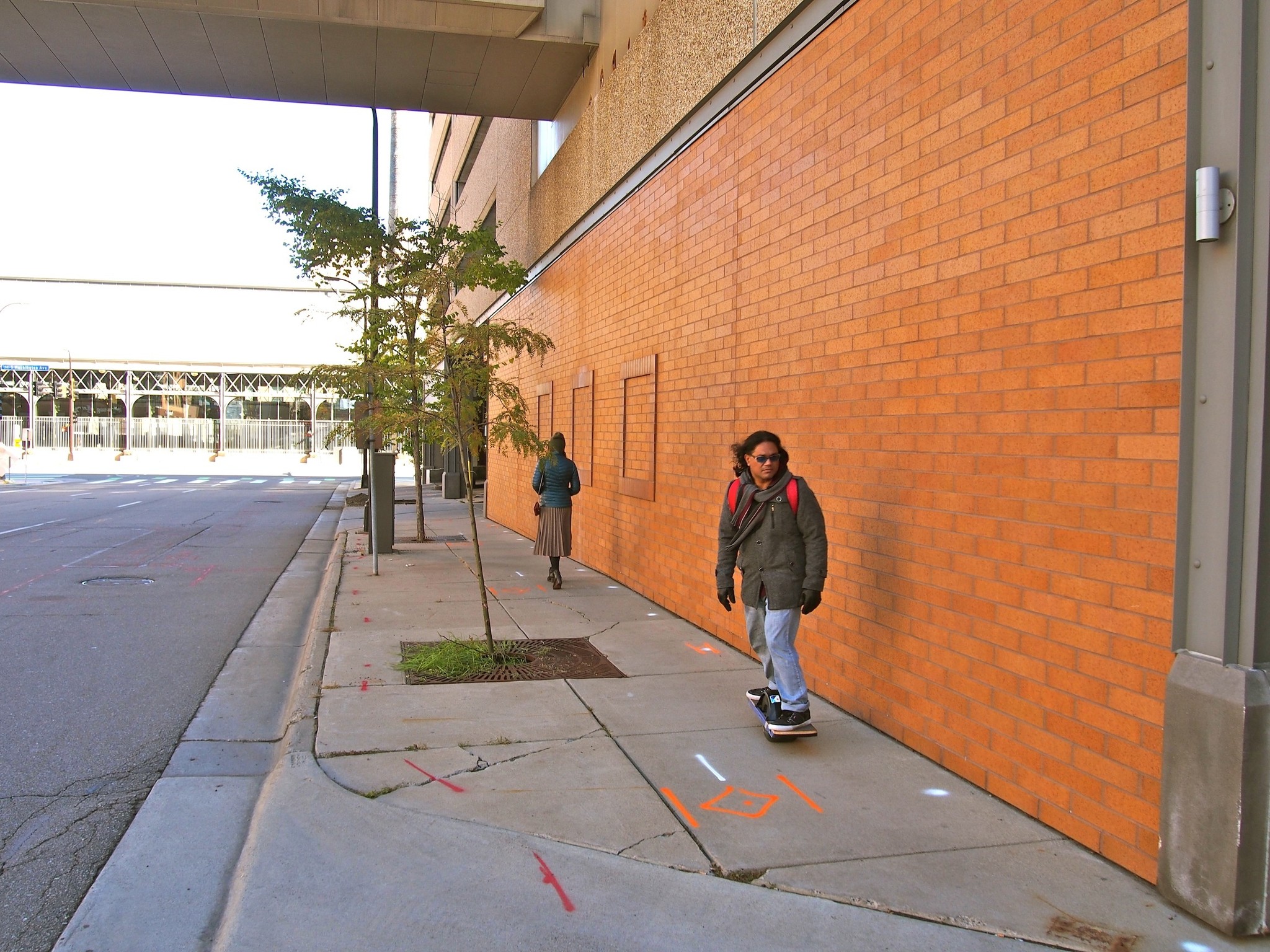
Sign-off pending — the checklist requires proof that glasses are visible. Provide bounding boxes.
[750,453,781,463]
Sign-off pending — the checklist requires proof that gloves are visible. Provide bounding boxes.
[718,587,736,611]
[798,590,821,614]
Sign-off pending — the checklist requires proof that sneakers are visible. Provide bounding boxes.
[746,687,778,701]
[768,708,812,730]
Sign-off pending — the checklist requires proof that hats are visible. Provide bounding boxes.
[549,432,565,451]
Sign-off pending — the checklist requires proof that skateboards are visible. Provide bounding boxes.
[746,688,819,744]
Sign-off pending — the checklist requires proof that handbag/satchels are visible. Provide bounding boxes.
[533,502,540,516]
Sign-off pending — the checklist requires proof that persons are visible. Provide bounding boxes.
[532,432,581,590]
[715,430,828,731]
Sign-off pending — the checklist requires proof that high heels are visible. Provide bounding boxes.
[553,570,562,589]
[548,567,555,582]
